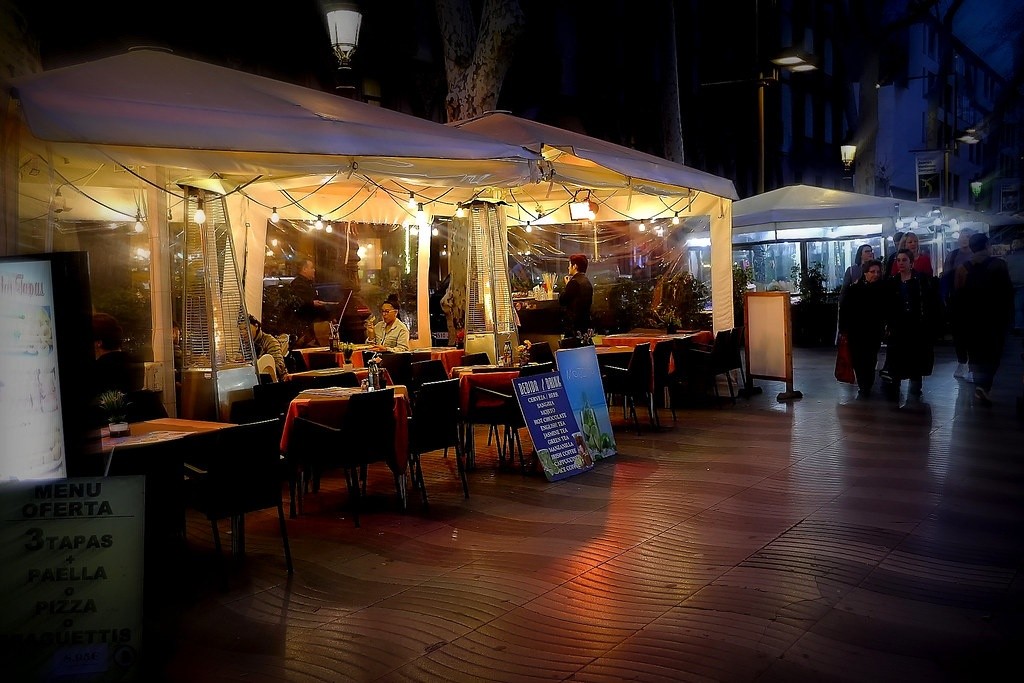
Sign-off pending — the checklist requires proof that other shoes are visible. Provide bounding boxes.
[967,372,975,383]
[855,361,923,411]
[954,364,969,378]
[974,385,993,403]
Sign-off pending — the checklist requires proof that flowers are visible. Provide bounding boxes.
[514,340,530,365]
[366,352,381,364]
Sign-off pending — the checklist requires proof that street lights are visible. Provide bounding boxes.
[876,71,958,204]
[756,45,821,194]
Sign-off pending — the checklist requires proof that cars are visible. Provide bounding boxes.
[312,283,372,341]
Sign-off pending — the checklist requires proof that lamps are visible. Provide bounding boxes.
[569,187,600,221]
[887,216,961,247]
[968,172,985,212]
[841,144,859,192]
[316,0,366,92]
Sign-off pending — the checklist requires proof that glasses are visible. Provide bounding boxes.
[379,310,396,314]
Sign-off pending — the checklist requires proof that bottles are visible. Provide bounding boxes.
[368,361,379,389]
[503,340,512,367]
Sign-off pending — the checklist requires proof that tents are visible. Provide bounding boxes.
[223,109,738,386]
[763,193,1024,274]
[684,184,899,283]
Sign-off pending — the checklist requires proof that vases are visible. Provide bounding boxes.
[367,363,381,390]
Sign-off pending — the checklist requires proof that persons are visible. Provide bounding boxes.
[1001,238,1024,338]
[839,244,875,295]
[950,232,1016,400]
[365,300,410,354]
[891,233,933,275]
[886,232,904,271]
[881,249,940,399]
[943,228,978,382]
[839,259,889,401]
[131,318,191,409]
[92,312,137,404]
[558,253,593,334]
[235,311,288,411]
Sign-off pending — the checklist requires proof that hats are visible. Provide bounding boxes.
[381,294,400,310]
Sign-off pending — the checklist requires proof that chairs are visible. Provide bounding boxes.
[343,387,406,528]
[459,351,489,366]
[681,329,733,410]
[558,336,579,350]
[648,340,677,425]
[710,326,746,401]
[408,359,448,383]
[192,417,294,574]
[410,377,469,511]
[602,341,654,434]
[525,341,557,365]
[253,381,299,417]
[507,361,556,463]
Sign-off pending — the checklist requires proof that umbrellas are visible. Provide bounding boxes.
[0,47,549,419]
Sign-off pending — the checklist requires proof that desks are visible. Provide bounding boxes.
[595,344,634,420]
[284,367,393,389]
[290,344,381,367]
[364,346,465,384]
[444,364,520,461]
[98,417,238,476]
[281,384,409,519]
[603,332,711,374]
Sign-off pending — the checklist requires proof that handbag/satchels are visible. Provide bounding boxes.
[835,332,855,384]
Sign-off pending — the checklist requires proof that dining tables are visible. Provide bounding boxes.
[313,372,359,387]
